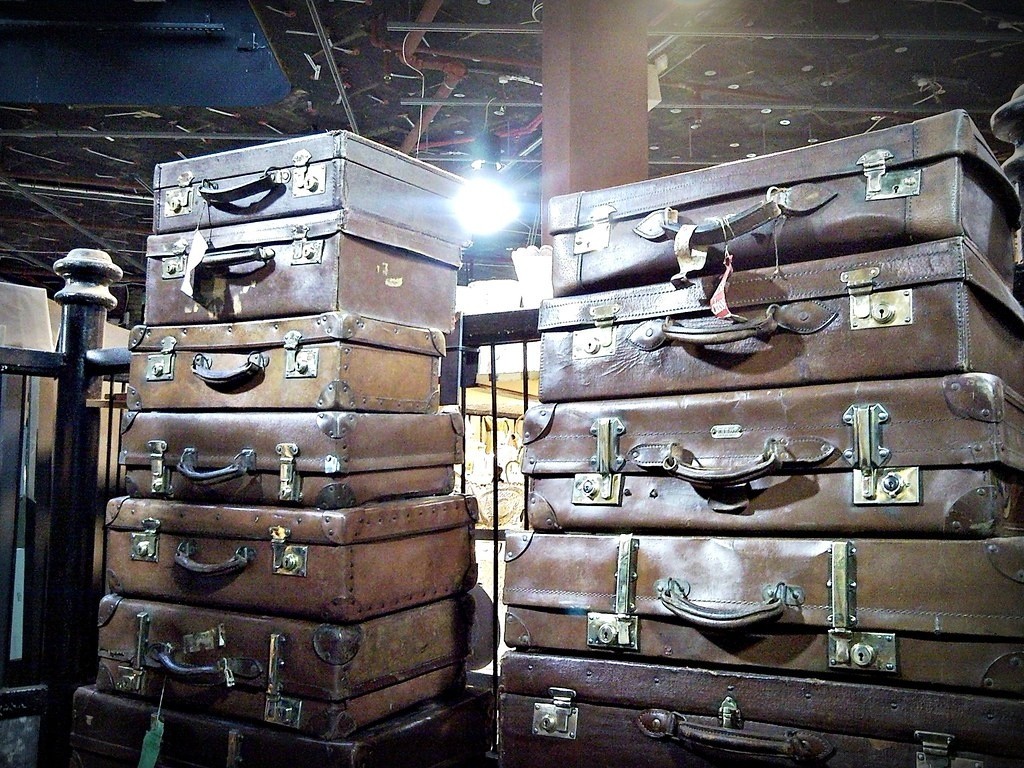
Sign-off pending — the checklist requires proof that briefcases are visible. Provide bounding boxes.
[66,129,497,768]
[497,108,1023,767]
